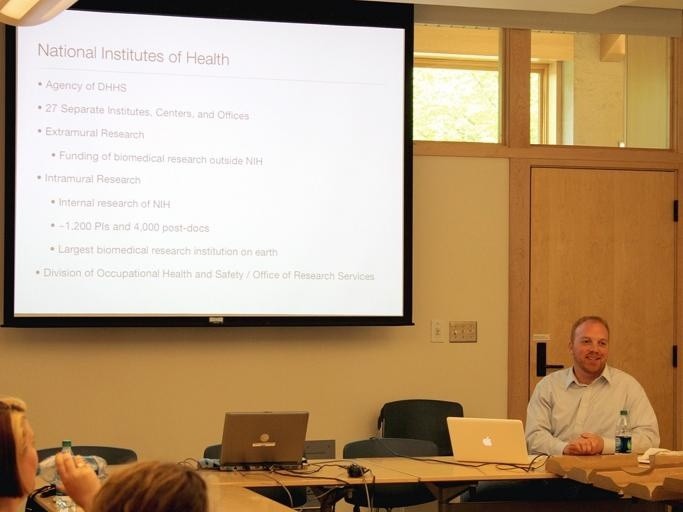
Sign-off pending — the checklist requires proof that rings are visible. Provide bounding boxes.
[76,463,82,468]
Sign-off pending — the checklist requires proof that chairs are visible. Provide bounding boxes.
[37,447,138,469]
[343,438,439,508]
[383,399,463,457]
[204,445,235,461]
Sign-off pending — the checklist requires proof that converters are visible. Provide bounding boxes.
[347,466,364,478]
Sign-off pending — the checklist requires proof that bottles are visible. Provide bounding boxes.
[614,409,633,457]
[34,439,108,512]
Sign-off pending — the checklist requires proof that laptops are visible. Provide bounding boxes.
[446,416,546,465]
[198,411,309,471]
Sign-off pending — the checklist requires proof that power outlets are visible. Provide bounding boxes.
[449,321,477,342]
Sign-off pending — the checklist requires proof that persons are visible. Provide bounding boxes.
[0,395,102,512]
[89,460,207,512]
[525,316,660,455]
